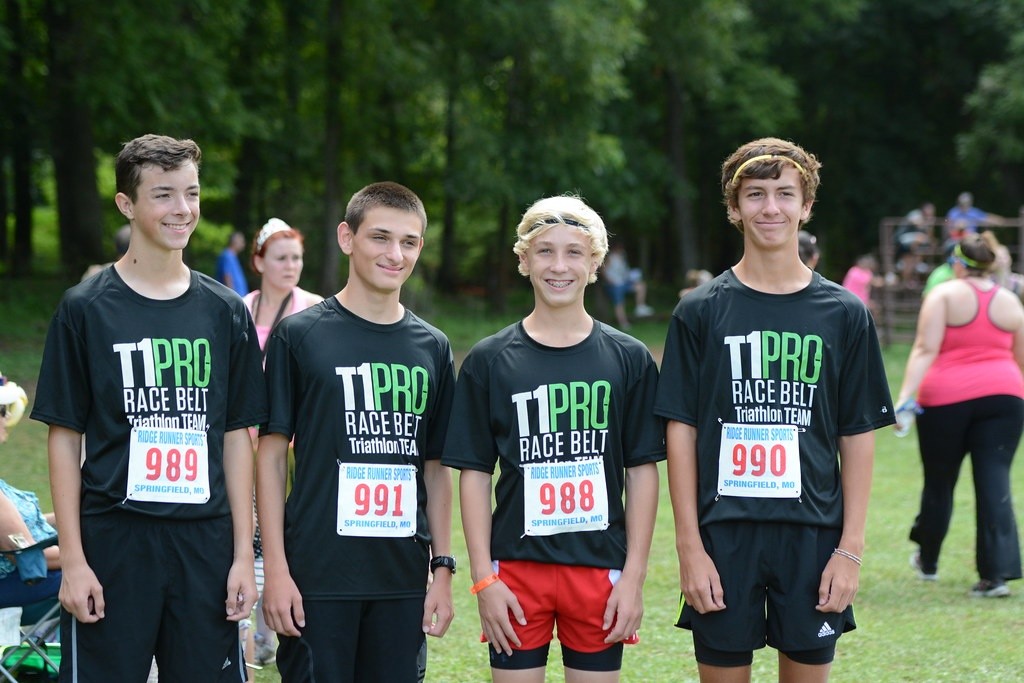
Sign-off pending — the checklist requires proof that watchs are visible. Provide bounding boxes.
[431,555,456,574]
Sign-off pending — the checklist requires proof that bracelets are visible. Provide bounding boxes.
[834,548,862,566]
[470,573,499,594]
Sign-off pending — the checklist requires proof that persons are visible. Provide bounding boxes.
[81,225,131,281]
[604,244,653,331]
[893,236,1024,596]
[257,182,456,683]
[217,232,249,298]
[440,195,666,683]
[0,371,63,606]
[842,194,1024,328]
[238,218,328,664]
[798,231,820,269]
[679,268,713,299]
[28,134,269,683]
[654,137,896,683]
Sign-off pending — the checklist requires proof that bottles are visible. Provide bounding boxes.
[893,401,916,438]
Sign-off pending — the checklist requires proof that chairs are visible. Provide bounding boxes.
[0,535,78,683]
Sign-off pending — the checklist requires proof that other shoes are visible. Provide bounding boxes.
[967,575,1013,597]
[910,549,937,580]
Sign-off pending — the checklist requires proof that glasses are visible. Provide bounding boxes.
[0,404,14,418]
